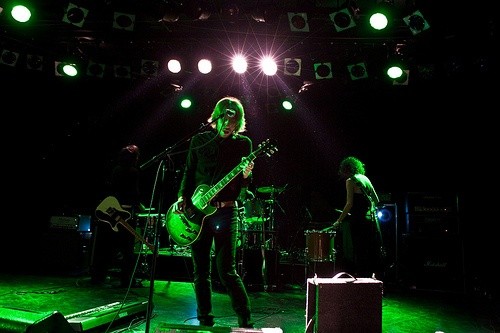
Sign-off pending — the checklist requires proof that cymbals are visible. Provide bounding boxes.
[258,186,282,193]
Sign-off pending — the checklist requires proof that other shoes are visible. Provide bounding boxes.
[121,281,140,288]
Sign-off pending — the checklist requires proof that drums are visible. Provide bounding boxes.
[305,231,337,263]
[134,213,166,228]
[211,218,263,253]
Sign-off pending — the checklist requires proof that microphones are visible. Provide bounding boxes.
[226,109,236,117]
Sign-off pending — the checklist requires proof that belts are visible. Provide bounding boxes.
[215,201,238,208]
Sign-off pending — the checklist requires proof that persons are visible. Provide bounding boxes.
[322,156,386,278]
[172,97,254,328]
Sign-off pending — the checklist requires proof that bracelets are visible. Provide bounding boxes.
[333,221,342,227]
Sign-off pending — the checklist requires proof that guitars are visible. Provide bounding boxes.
[95,196,159,255]
[165,138,280,246]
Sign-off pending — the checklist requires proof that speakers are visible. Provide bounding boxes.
[306,277,383,333]
[0,307,76,333]
